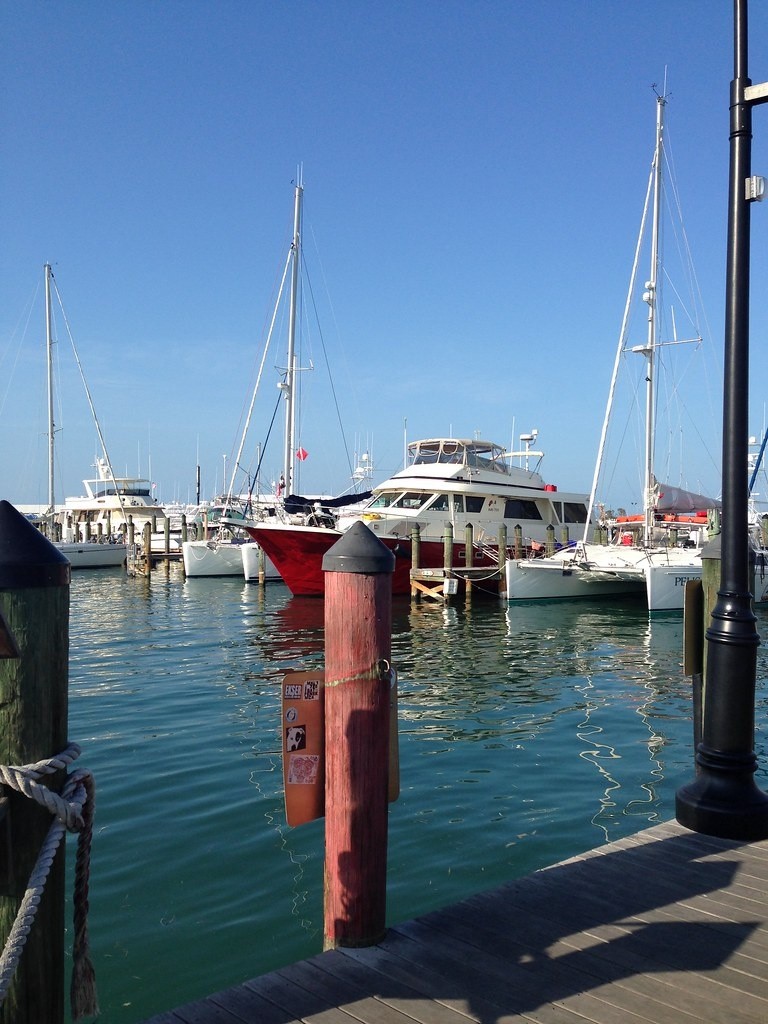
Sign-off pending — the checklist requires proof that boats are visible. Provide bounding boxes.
[218,417,600,596]
[65,454,180,551]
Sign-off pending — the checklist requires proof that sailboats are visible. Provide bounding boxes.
[182,161,375,584]
[506,50,768,612]
[19,261,130,567]
